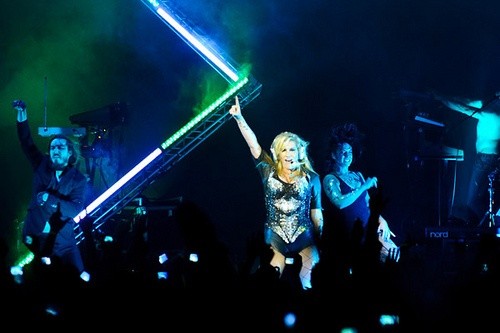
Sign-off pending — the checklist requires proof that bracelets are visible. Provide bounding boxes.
[233,113,242,119]
[41,192,49,203]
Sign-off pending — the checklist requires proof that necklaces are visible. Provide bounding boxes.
[283,165,300,177]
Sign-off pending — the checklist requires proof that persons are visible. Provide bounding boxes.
[256,214,499,281]
[323,122,396,273]
[152,201,243,333]
[229,94,324,291]
[484,139,500,214]
[12,101,88,277]
[0,194,170,333]
